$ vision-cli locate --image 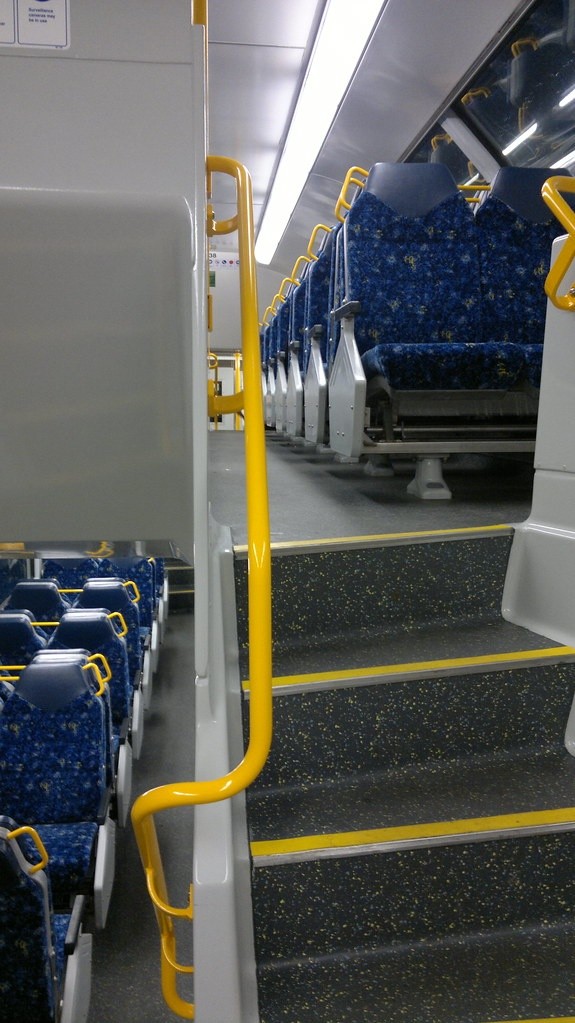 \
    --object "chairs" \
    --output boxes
[240,164,575,504]
[0,557,169,1023]
